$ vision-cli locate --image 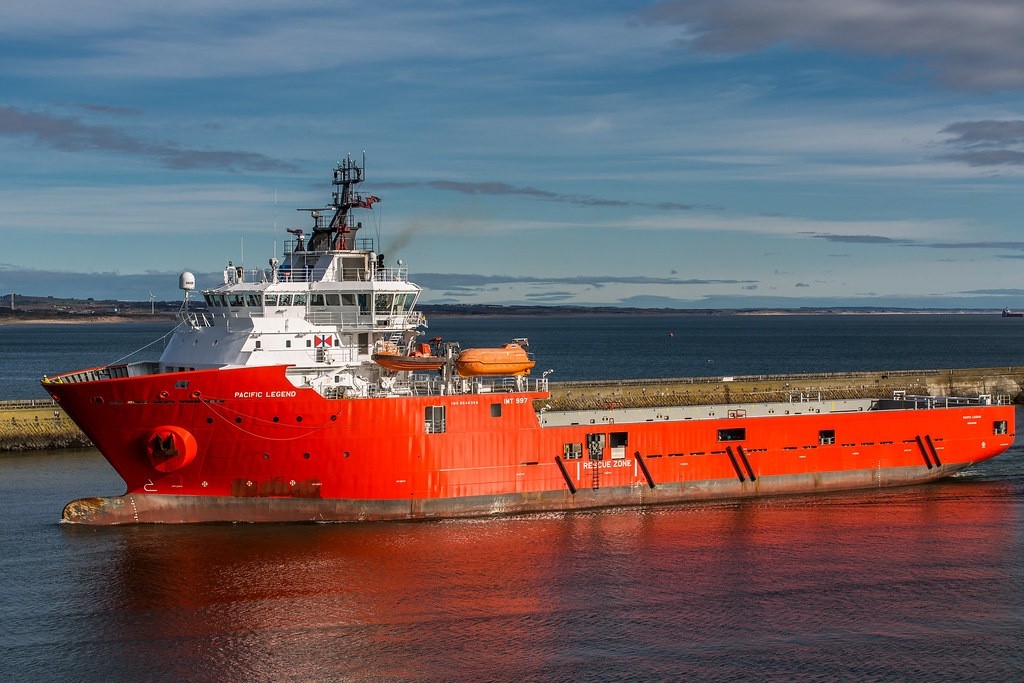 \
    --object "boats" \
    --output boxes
[1001,307,1024,317]
[453,339,537,378]
[41,146,1017,526]
[369,342,445,372]
